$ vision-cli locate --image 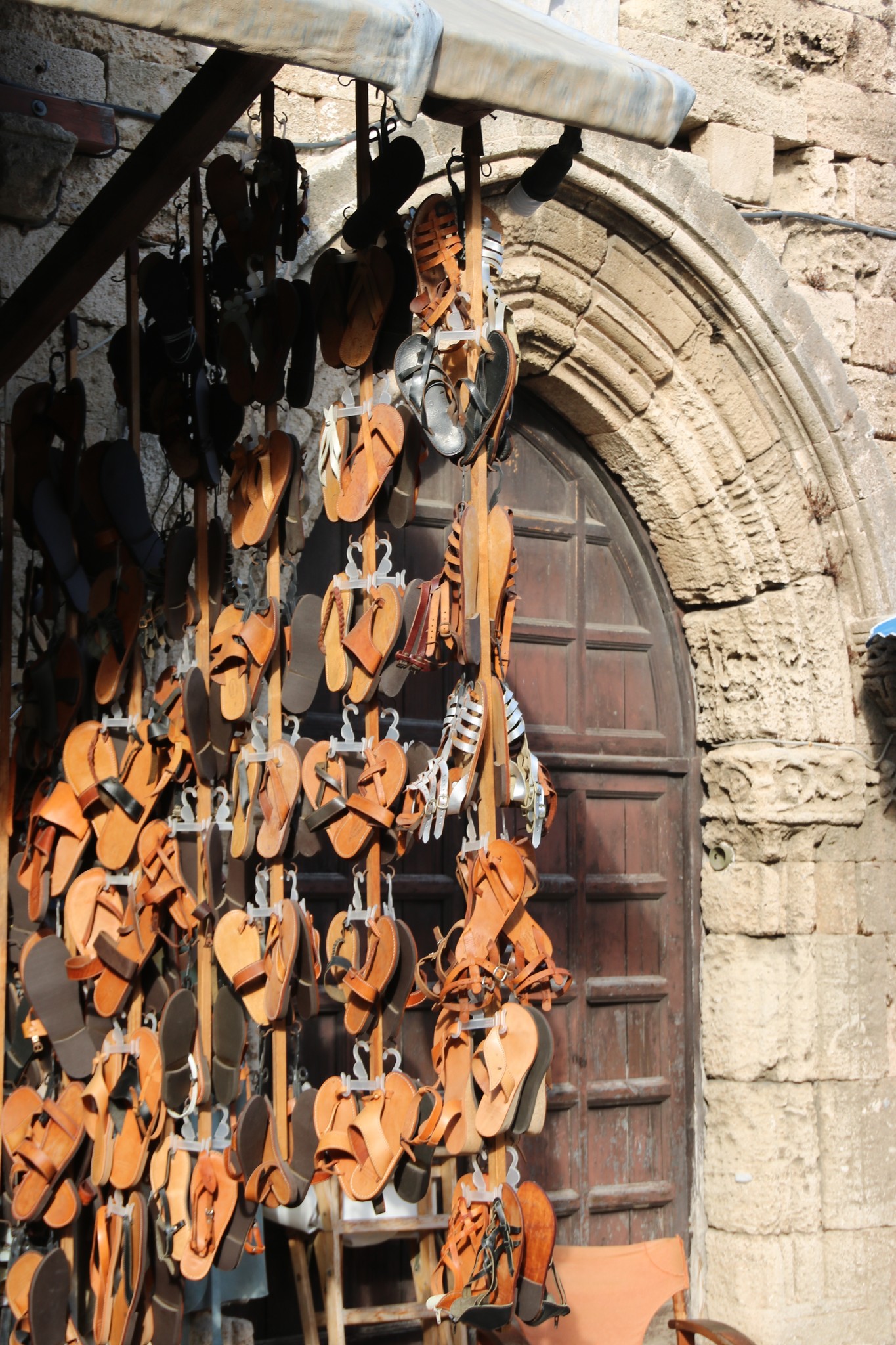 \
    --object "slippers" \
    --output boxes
[0,143,570,1344]
